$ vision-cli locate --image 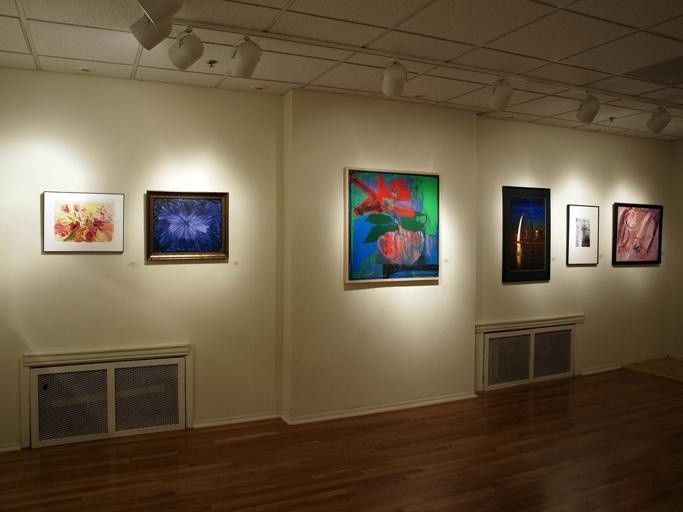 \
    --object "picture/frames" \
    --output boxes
[502,186,551,285]
[566,204,600,267]
[343,167,444,287]
[146,190,230,263]
[42,190,125,255]
[612,202,663,267]
